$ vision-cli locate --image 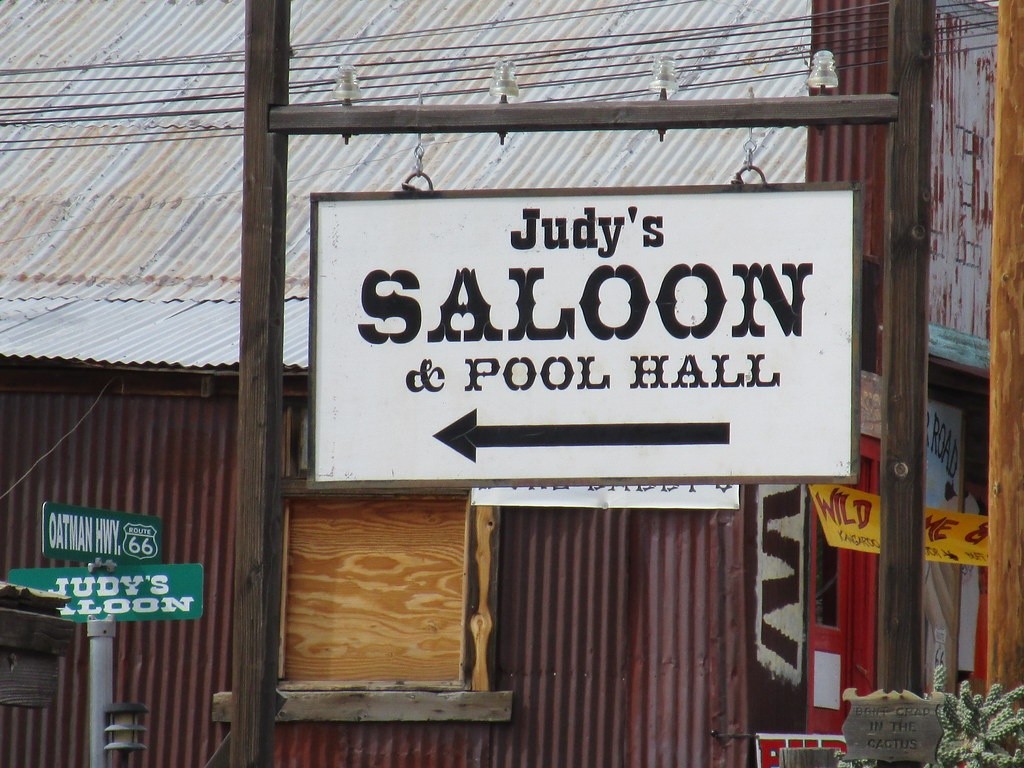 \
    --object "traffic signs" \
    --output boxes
[5,563,204,622]
[41,502,162,565]
[311,185,863,489]
